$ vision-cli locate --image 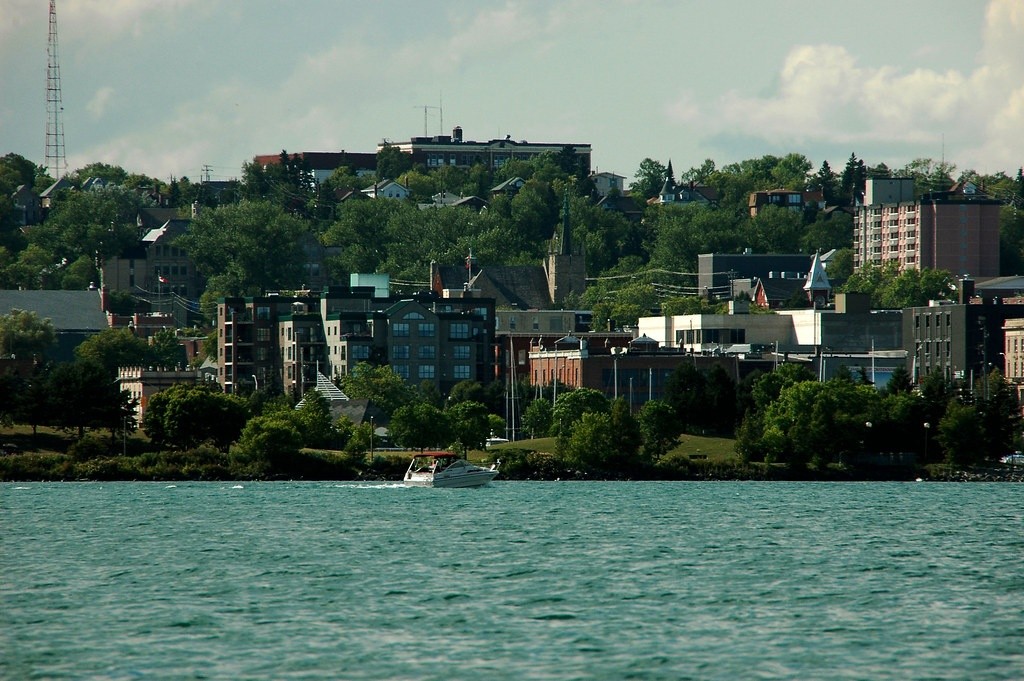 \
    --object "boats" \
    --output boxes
[403,452,499,489]
